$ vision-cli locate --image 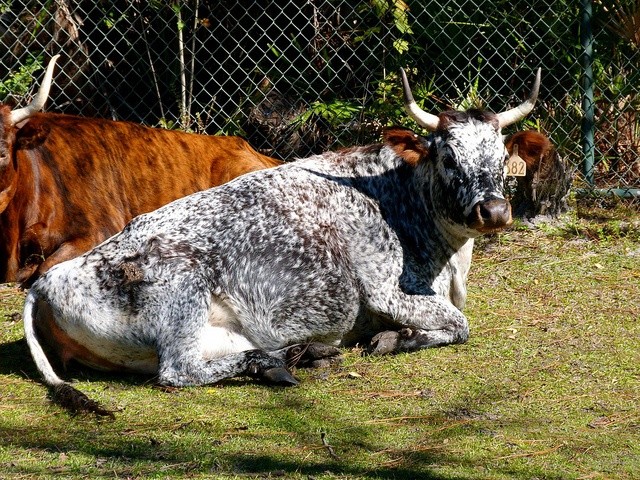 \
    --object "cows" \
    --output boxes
[23,66,556,425]
[1,53,282,290]
